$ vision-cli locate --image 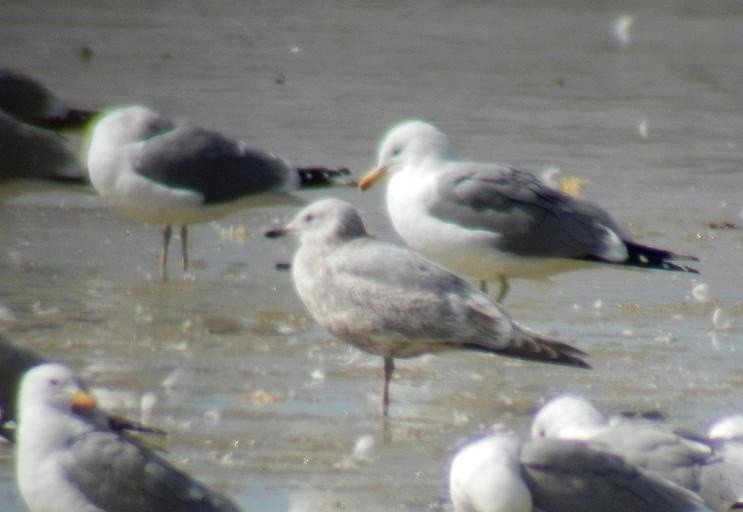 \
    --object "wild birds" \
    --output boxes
[446,394,743,511]
[0,67,102,132]
[12,361,243,511]
[0,109,89,183]
[263,197,591,405]
[0,334,166,442]
[85,102,358,287]
[359,119,703,307]
